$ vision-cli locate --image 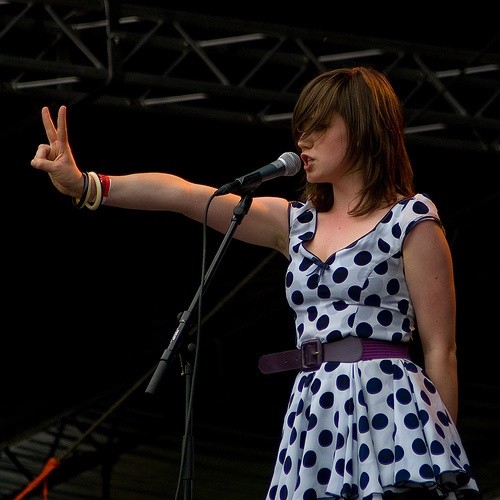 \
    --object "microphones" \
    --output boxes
[215,152,301,196]
